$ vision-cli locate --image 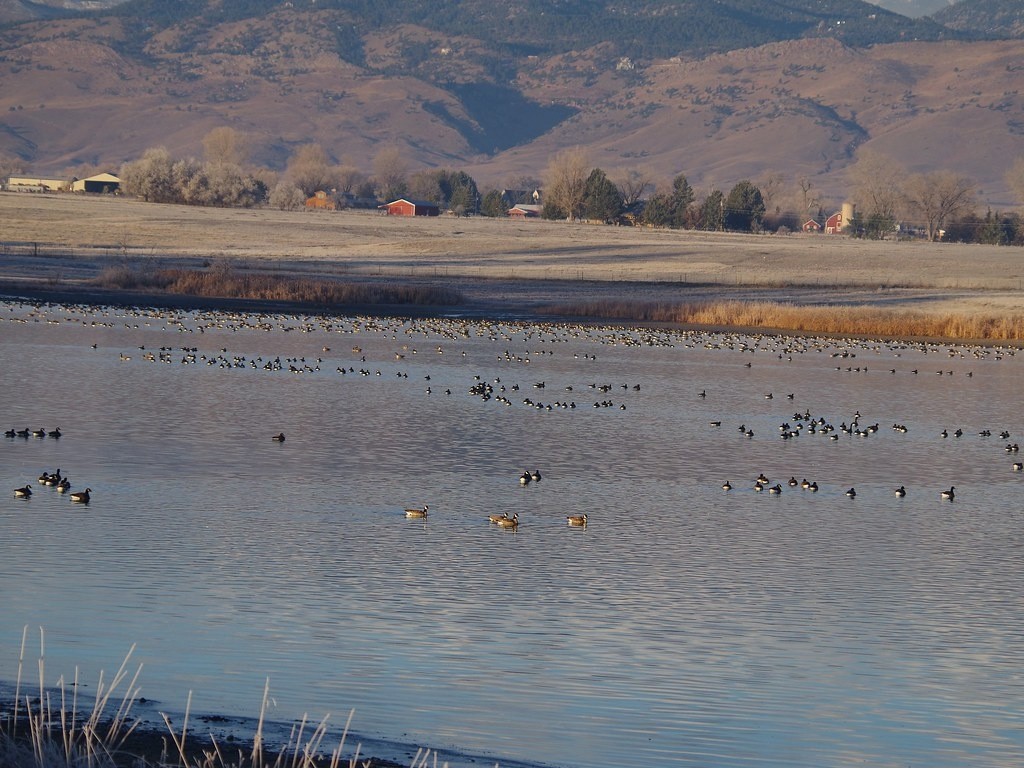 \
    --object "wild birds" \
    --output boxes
[14,485,31,496]
[0,296,1024,470]
[757,474,769,484]
[754,482,763,492]
[70,488,92,503]
[488,513,508,522]
[567,514,587,524]
[39,469,70,493]
[810,482,818,491]
[404,506,428,516]
[520,471,531,484]
[942,486,956,498]
[532,470,541,482]
[770,484,781,493]
[496,513,519,527]
[723,480,731,490]
[896,486,906,496]
[789,477,798,486]
[847,488,856,497]
[801,479,810,488]
